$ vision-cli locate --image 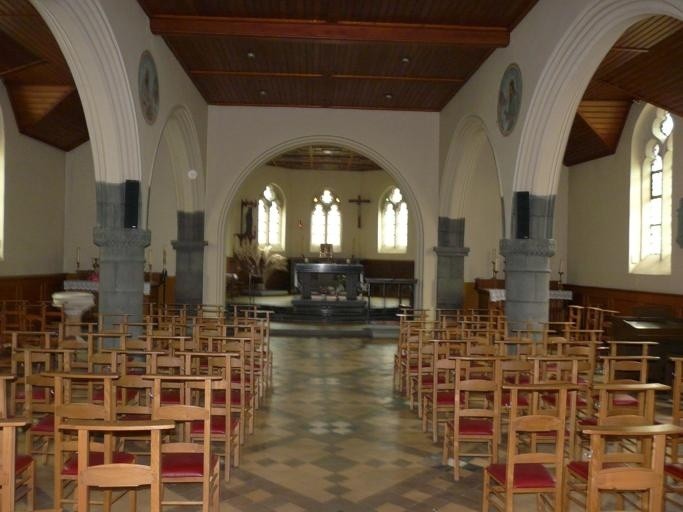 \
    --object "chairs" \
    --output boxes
[0,417,31,512]
[582,423,682,511]
[481,381,575,511]
[40,370,118,512]
[142,374,223,512]
[58,417,177,512]
